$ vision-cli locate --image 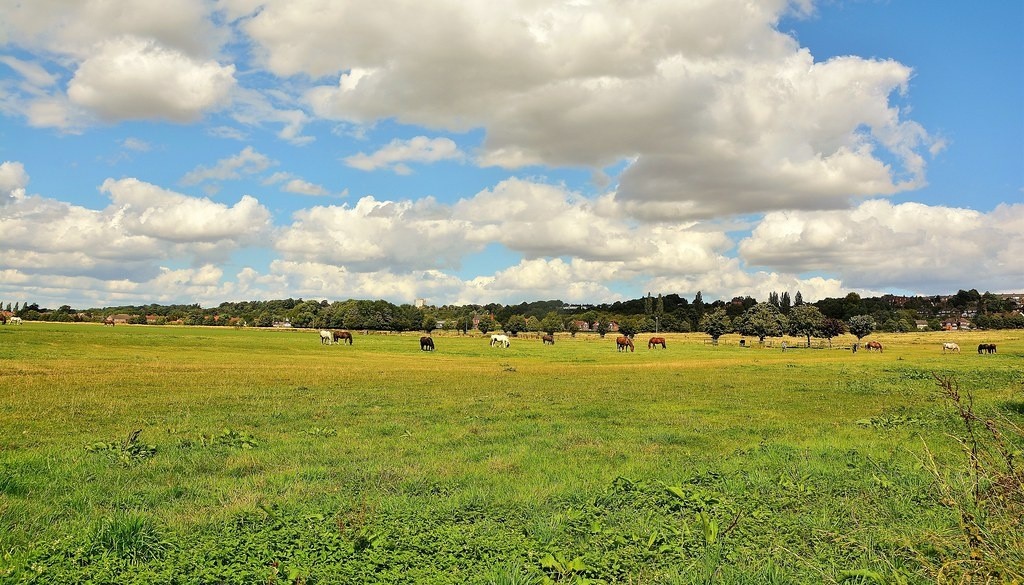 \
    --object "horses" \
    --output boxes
[976,344,996,355]
[781,341,786,351]
[420,337,434,351]
[489,334,510,348]
[648,337,667,350]
[852,344,858,354]
[865,340,884,354]
[738,340,745,346]
[320,330,332,344]
[942,342,961,355]
[542,334,555,345]
[333,330,353,346]
[616,336,634,353]
